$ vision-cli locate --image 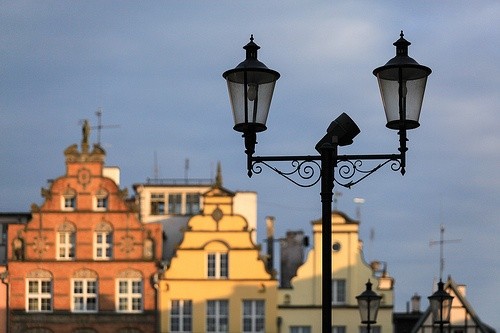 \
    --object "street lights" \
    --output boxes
[222,29,433,333]
[354,276,455,333]
